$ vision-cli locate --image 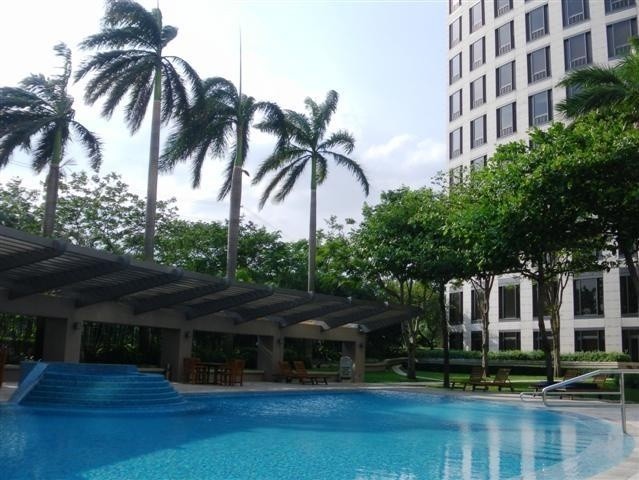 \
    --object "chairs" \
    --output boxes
[529,369,577,398]
[449,367,488,392]
[182,356,342,388]
[481,367,515,392]
[555,373,609,401]
